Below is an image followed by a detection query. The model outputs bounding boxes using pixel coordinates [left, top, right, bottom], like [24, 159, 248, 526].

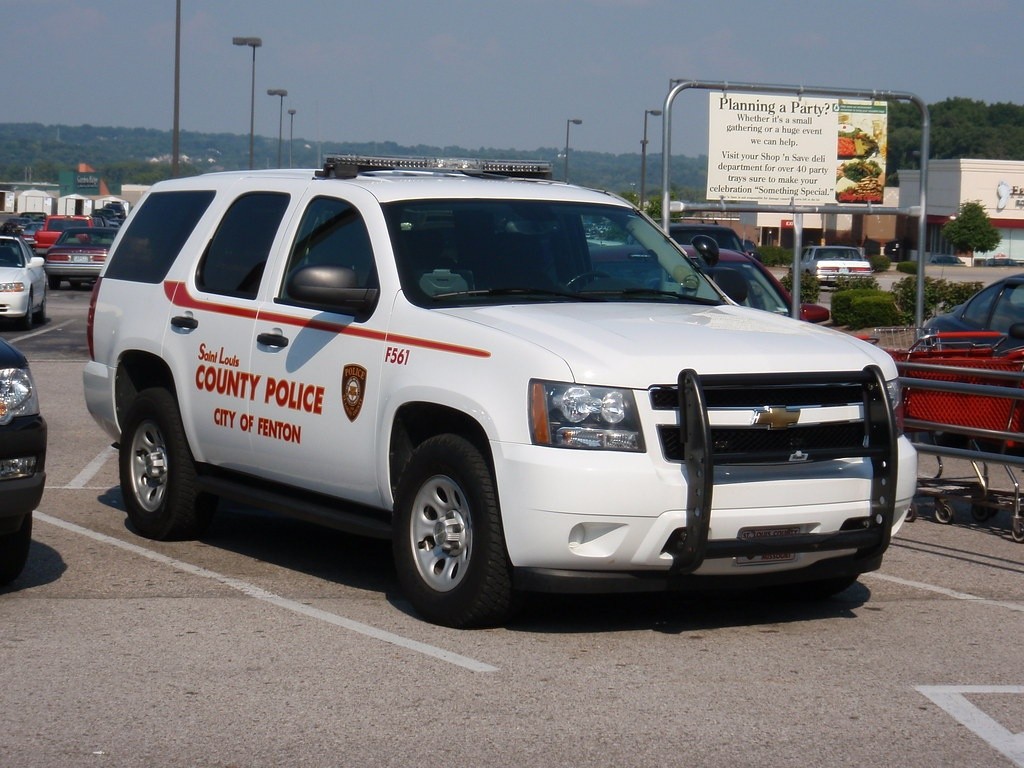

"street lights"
[564, 119, 582, 182]
[288, 109, 297, 169]
[640, 109, 662, 211]
[233, 37, 262, 170]
[267, 89, 288, 169]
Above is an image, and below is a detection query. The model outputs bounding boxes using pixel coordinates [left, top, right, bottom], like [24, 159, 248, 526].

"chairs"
[495, 234, 577, 293]
[67, 238, 81, 244]
[0, 247, 16, 261]
[366, 230, 476, 295]
[101, 239, 113, 244]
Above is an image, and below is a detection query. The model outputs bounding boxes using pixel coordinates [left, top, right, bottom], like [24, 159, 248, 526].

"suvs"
[83, 162, 917, 629]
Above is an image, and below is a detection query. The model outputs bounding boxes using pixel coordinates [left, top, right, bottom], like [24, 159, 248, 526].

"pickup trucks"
[32, 215, 93, 254]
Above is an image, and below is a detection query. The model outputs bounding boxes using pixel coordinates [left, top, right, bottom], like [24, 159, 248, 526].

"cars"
[0, 337, 48, 584]
[924, 273, 1024, 357]
[89, 203, 127, 228]
[929, 255, 967, 267]
[984, 259, 1017, 267]
[789, 246, 873, 288]
[624, 224, 755, 257]
[44, 227, 119, 290]
[0, 236, 49, 330]
[588, 244, 830, 323]
[0, 212, 48, 244]
